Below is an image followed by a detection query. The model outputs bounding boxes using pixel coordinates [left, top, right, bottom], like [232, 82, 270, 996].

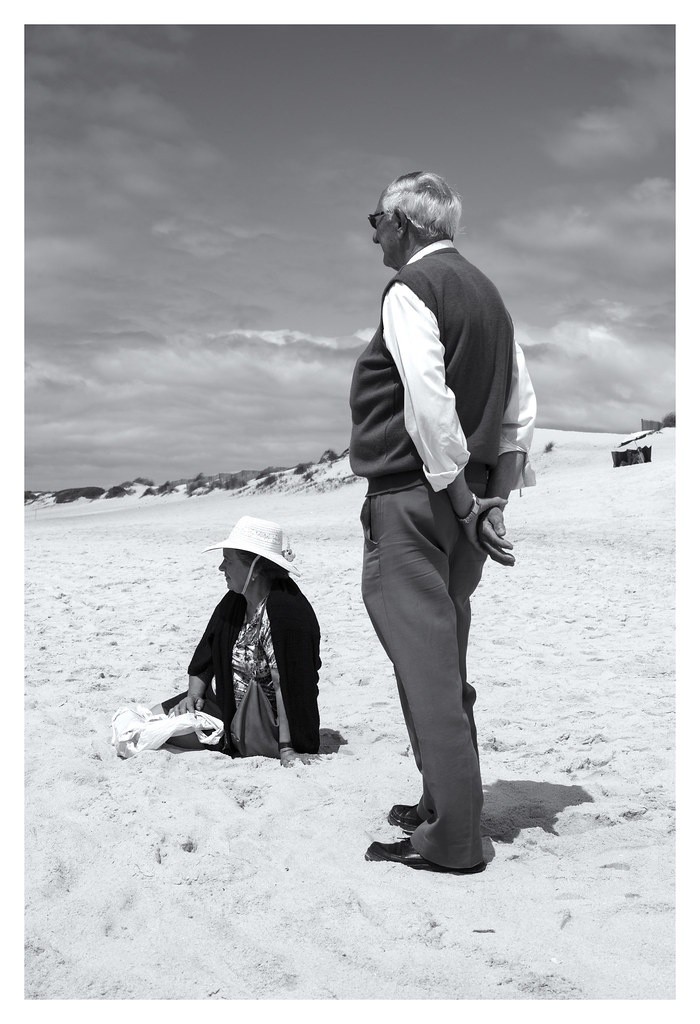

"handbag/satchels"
[231, 679, 281, 758]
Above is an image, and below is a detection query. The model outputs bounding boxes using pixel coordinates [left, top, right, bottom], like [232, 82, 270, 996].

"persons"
[151, 515, 322, 769]
[348, 168, 537, 875]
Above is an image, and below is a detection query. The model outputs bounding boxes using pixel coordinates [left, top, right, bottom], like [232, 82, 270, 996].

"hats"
[201, 514, 302, 577]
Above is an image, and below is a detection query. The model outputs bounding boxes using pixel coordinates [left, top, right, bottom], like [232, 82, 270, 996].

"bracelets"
[278, 742, 294, 753]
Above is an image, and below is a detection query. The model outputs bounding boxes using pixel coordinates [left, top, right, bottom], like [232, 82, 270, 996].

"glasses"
[366, 211, 384, 229]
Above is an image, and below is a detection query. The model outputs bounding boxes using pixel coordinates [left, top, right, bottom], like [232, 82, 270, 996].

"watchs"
[458, 490, 481, 527]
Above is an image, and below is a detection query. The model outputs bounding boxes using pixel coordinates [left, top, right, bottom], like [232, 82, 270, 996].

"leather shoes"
[388, 804, 424, 832]
[366, 836, 486, 875]
